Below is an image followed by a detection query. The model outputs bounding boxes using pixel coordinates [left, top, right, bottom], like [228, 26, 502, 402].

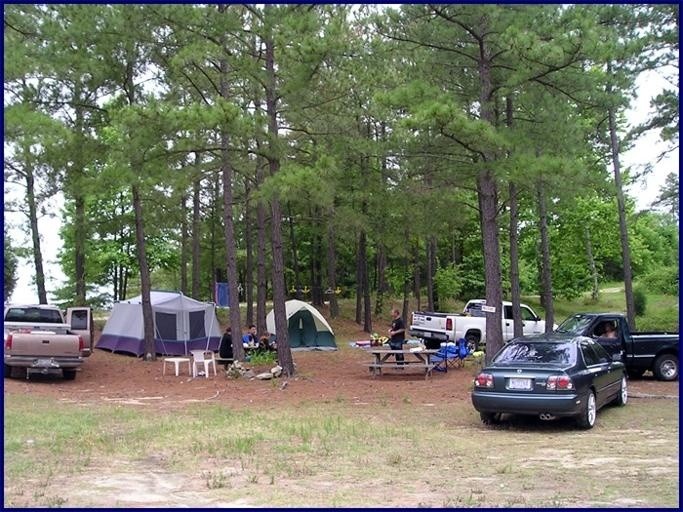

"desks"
[366, 349, 440, 377]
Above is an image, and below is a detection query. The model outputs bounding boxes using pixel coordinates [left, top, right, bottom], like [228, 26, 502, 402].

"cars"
[471, 332, 630, 429]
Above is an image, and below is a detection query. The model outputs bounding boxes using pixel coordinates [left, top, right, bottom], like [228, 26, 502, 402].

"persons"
[592, 322, 618, 338]
[242, 325, 278, 353]
[219, 327, 234, 371]
[388, 309, 406, 370]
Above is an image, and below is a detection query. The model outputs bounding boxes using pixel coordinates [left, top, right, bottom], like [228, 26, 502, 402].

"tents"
[94, 290, 223, 358]
[266, 299, 338, 352]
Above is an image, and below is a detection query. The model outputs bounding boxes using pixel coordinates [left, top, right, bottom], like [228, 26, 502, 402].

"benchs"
[214, 357, 233, 370]
[362, 360, 436, 381]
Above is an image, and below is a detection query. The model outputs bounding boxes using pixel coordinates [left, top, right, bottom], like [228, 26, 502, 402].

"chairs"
[188, 349, 217, 379]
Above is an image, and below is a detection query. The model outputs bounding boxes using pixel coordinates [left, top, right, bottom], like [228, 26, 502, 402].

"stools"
[162, 357, 190, 376]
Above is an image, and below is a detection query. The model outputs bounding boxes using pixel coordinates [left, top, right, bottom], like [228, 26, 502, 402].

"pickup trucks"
[408, 297, 560, 354]
[543, 311, 680, 382]
[3, 303, 94, 382]
